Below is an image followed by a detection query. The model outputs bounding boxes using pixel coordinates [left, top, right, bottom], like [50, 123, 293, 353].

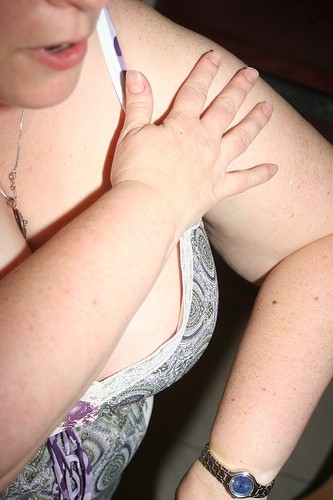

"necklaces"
[0, 109, 27, 241]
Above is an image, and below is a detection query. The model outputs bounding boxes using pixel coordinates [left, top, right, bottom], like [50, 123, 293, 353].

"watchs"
[197, 443, 276, 500]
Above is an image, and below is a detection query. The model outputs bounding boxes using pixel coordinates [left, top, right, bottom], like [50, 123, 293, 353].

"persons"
[0, 1, 332, 500]
